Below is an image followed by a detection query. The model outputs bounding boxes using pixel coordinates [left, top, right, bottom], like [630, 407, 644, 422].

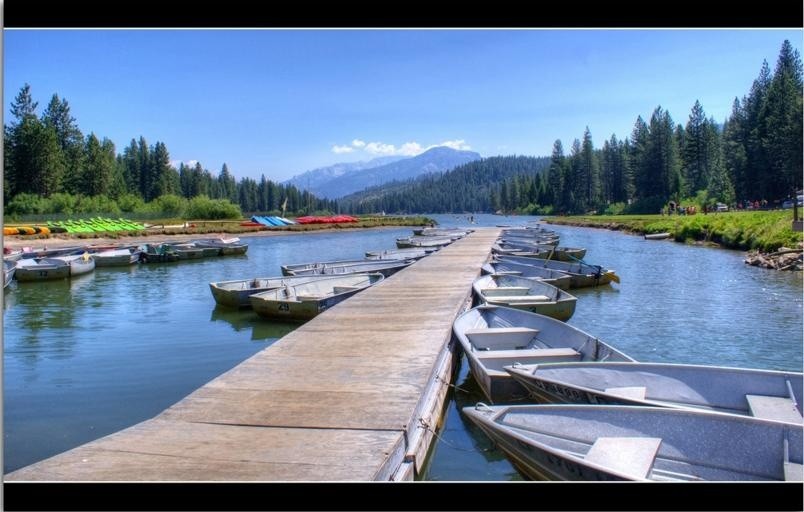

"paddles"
[567, 253, 620, 284]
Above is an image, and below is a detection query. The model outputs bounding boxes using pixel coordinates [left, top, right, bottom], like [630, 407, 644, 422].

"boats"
[1, 236, 249, 290]
[4, 216, 146, 238]
[249, 214, 358, 228]
[209, 226, 475, 320]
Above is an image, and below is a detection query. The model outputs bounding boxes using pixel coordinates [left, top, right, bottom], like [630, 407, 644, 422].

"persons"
[660, 198, 770, 217]
